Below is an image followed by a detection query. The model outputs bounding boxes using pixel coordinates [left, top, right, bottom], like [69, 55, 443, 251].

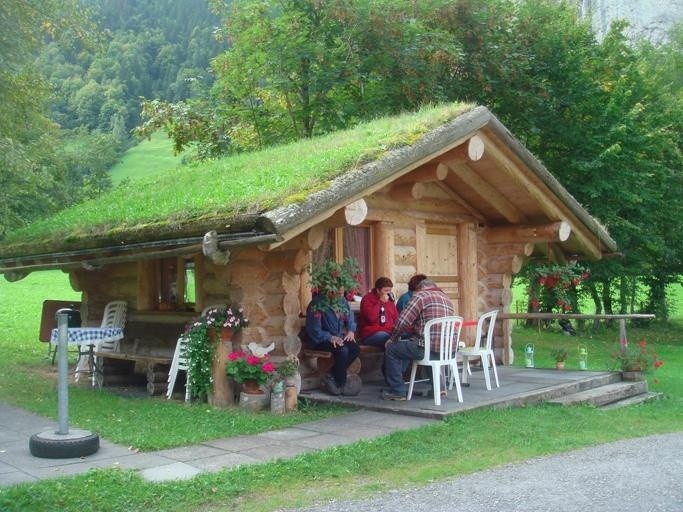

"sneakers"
[382, 390, 407, 401]
[427, 390, 447, 399]
[320, 373, 344, 394]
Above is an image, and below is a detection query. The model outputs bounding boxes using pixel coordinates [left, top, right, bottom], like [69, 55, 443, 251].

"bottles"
[378, 303, 385, 326]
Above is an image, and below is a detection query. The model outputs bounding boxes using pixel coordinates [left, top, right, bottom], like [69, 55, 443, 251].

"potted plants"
[549, 347, 569, 370]
[276, 356, 304, 387]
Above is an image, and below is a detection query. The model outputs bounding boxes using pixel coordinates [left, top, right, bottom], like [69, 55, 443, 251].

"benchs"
[302, 344, 384, 360]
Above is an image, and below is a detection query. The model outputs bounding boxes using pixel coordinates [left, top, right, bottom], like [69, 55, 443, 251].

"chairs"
[406, 315, 465, 407]
[164, 302, 228, 403]
[447, 309, 501, 392]
[73, 299, 129, 387]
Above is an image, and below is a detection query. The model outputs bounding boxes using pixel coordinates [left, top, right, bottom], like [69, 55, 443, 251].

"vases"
[207, 327, 235, 341]
[620, 370, 645, 382]
[241, 379, 260, 394]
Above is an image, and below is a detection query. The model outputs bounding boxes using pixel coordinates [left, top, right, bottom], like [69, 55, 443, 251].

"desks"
[49, 327, 126, 374]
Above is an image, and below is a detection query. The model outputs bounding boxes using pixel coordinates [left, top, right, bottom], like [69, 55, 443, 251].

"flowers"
[608, 338, 663, 386]
[301, 254, 365, 321]
[224, 351, 275, 386]
[177, 302, 251, 406]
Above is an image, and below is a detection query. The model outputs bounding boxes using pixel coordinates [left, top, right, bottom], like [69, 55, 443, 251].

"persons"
[359, 277, 415, 386]
[305, 284, 360, 395]
[396, 275, 427, 341]
[382, 279, 457, 401]
